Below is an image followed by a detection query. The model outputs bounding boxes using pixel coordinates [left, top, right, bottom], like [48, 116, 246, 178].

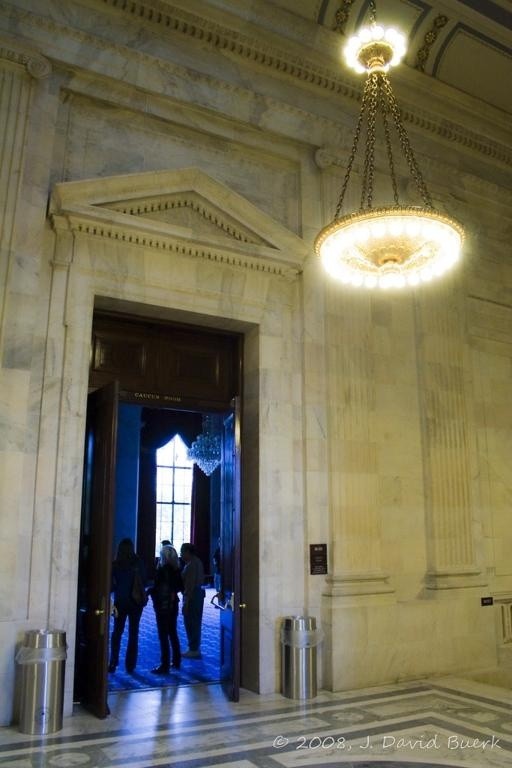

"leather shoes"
[152, 666, 169, 673]
[110, 663, 115, 671]
[125, 662, 135, 671]
[170, 662, 180, 669]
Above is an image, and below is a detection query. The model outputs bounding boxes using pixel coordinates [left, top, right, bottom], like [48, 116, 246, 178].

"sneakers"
[182, 650, 201, 657]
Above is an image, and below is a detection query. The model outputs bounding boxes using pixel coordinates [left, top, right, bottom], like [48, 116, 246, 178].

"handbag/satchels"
[132, 582, 143, 600]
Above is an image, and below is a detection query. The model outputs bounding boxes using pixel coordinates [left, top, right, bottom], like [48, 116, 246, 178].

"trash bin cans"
[16, 629, 66, 735]
[282, 616, 318, 700]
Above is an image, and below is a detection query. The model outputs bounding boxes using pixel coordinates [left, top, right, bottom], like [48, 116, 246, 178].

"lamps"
[187, 413, 220, 476]
[308, 0, 470, 294]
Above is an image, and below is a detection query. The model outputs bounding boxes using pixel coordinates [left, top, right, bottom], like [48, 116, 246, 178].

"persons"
[180, 543, 204, 659]
[156, 540, 173, 570]
[110, 538, 149, 673]
[147, 545, 184, 673]
